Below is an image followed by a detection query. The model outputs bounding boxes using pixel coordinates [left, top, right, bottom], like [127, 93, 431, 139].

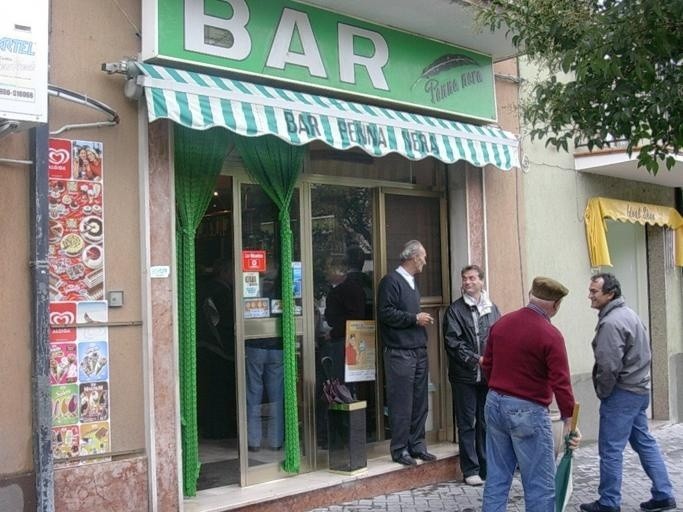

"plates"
[47, 178, 103, 301]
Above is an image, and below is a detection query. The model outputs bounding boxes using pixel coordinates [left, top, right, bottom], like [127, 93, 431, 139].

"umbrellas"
[552, 400, 580, 512]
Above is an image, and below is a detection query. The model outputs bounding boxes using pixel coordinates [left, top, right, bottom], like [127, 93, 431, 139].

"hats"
[532, 277, 568, 300]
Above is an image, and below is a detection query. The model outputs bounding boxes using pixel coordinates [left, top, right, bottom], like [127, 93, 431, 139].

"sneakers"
[580, 501, 619, 512]
[640, 499, 676, 511]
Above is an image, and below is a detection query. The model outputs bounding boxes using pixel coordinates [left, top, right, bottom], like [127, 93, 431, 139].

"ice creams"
[79, 344, 107, 377]
[48, 347, 74, 384]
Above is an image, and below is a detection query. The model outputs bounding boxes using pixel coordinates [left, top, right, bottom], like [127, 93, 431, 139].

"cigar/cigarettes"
[430, 320, 433, 325]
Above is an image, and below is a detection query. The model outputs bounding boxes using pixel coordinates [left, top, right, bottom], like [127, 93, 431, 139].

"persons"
[482, 276, 582, 512]
[207, 246, 374, 452]
[441, 264, 503, 486]
[376, 239, 437, 467]
[579, 272, 677, 512]
[73, 141, 101, 181]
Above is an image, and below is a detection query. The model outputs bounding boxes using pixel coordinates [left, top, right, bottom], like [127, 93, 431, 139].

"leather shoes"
[399, 456, 416, 465]
[464, 475, 482, 485]
[419, 453, 435, 461]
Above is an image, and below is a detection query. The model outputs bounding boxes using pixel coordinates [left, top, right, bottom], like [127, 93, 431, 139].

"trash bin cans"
[327, 399, 368, 476]
